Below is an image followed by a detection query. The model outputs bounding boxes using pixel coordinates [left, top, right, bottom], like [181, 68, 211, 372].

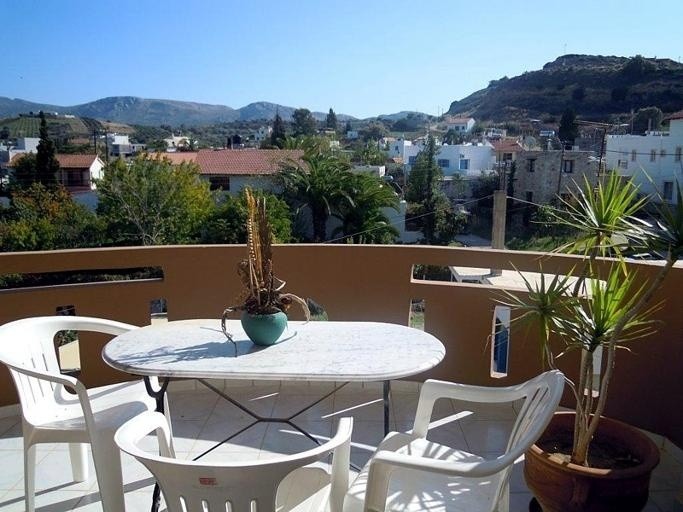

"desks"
[102, 318, 446, 512]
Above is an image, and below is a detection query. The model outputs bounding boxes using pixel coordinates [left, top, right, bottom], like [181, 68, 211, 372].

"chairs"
[0, 315, 176, 512]
[343, 370, 564, 512]
[113, 410, 353, 512]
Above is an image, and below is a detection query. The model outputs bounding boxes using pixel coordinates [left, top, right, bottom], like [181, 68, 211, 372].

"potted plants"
[222, 188, 310, 357]
[488, 161, 683, 512]
